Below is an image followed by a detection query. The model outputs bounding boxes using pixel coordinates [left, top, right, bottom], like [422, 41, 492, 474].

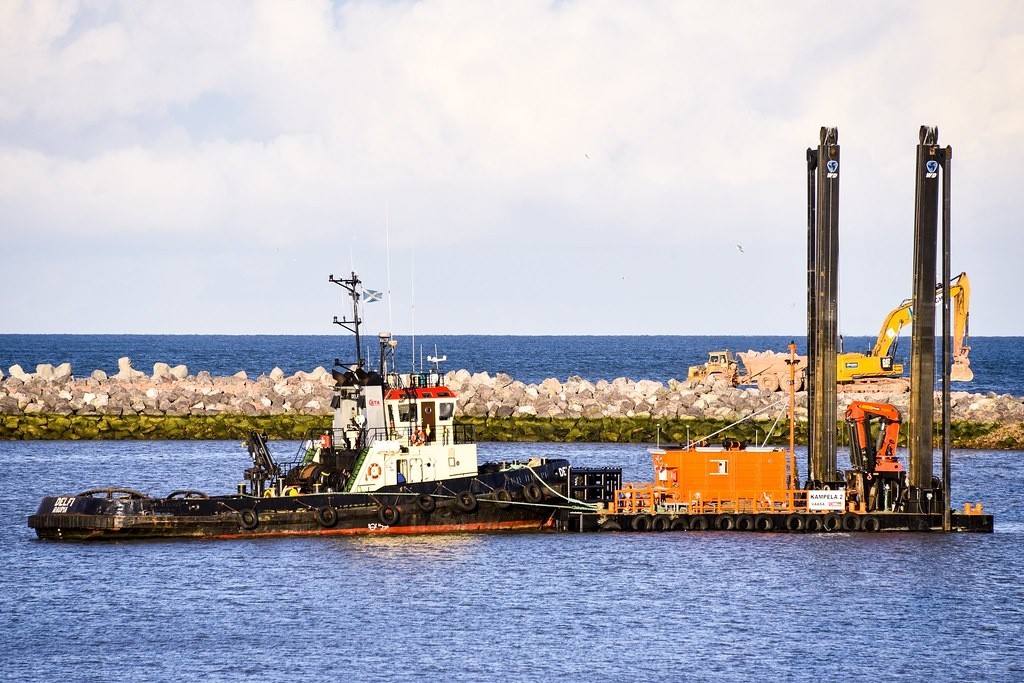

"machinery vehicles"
[836, 271, 974, 393]
[844, 400, 905, 505]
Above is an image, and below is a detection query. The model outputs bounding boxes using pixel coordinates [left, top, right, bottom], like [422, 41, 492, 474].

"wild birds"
[736, 243, 744, 254]
[585, 154, 589, 158]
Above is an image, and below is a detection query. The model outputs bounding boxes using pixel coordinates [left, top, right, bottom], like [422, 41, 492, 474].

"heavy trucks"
[687, 348, 807, 392]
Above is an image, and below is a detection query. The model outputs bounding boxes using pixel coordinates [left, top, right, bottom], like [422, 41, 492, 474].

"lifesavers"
[367, 463, 382, 479]
[410, 429, 426, 446]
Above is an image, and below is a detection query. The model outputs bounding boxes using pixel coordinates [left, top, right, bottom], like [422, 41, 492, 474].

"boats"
[24, 212, 574, 542]
